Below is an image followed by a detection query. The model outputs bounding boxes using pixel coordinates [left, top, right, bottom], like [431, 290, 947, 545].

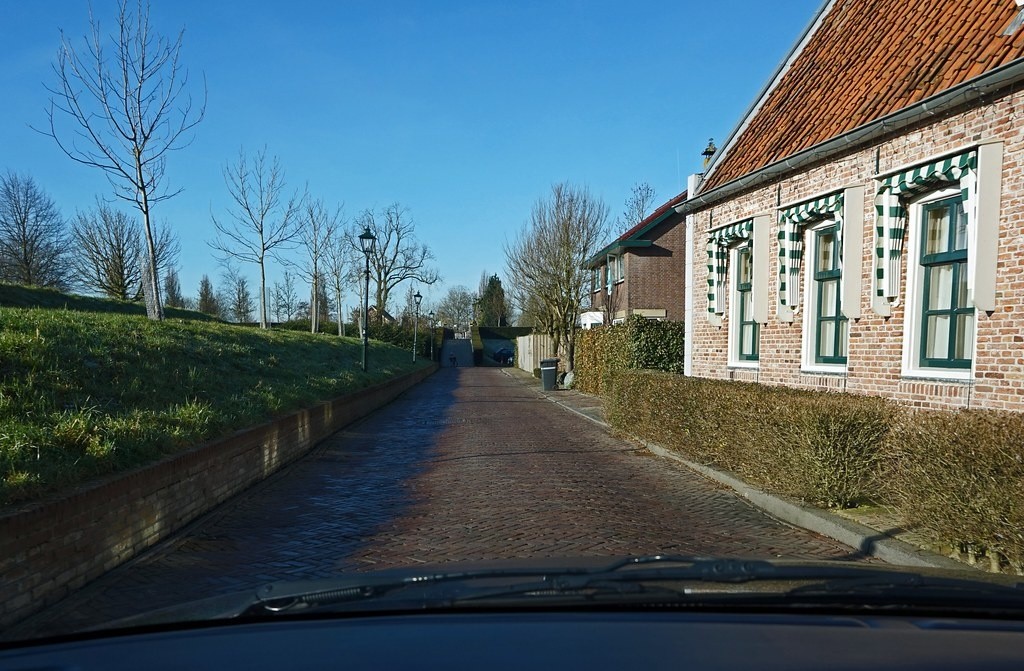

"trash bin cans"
[540, 358, 561, 391]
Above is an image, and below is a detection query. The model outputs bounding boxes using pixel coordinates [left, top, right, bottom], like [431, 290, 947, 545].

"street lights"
[412, 290, 422, 362]
[429, 310, 435, 361]
[358, 225, 376, 372]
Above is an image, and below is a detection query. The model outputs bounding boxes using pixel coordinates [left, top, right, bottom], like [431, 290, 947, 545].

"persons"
[448, 328, 454, 339]
[449, 353, 458, 368]
